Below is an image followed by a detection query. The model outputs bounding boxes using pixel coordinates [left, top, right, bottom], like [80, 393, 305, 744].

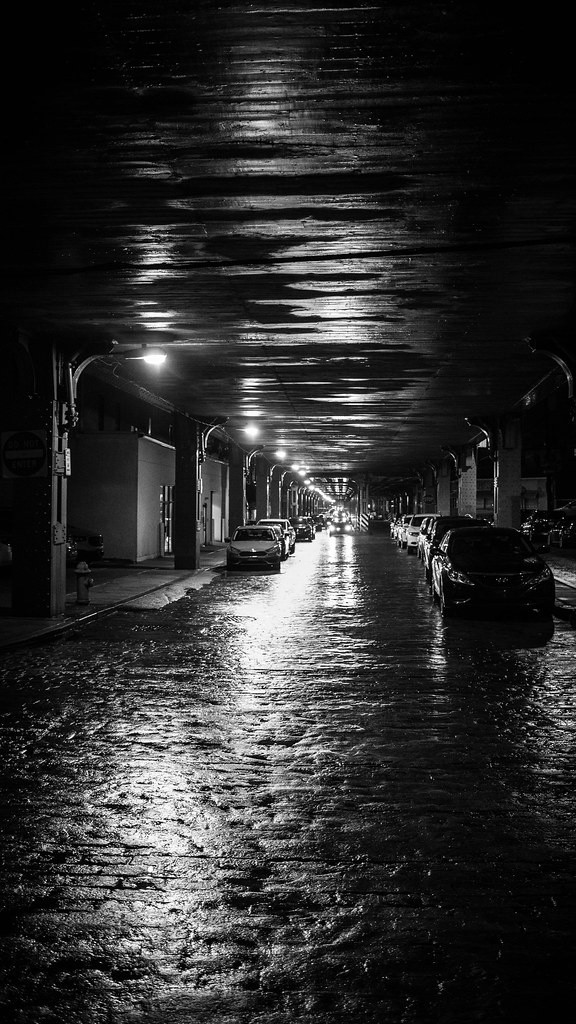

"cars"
[224, 525, 284, 571]
[289, 514, 328, 542]
[66, 524, 105, 567]
[256, 518, 298, 555]
[273, 524, 290, 561]
[430, 525, 556, 620]
[520, 500, 576, 547]
[388, 513, 493, 586]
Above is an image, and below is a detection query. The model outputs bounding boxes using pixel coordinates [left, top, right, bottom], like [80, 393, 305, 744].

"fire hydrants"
[74, 561, 94, 604]
